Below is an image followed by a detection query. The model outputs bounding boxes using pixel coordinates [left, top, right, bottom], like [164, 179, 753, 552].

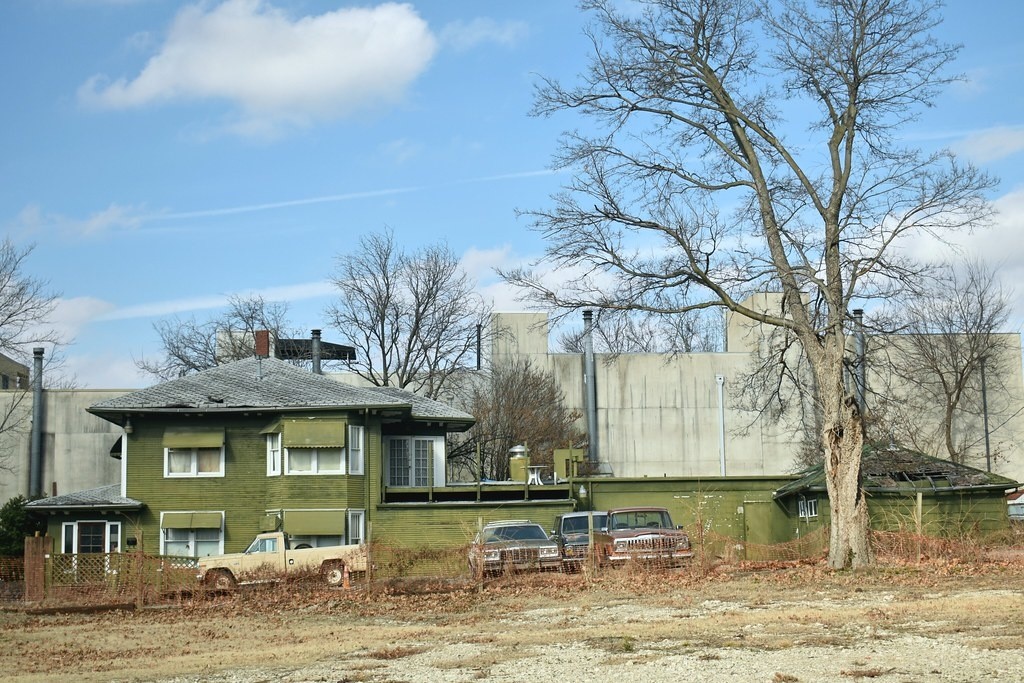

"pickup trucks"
[198, 531, 370, 594]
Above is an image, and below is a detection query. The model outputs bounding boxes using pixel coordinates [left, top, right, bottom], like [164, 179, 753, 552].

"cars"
[601, 507, 693, 566]
[550, 511, 607, 573]
[468, 518, 563, 578]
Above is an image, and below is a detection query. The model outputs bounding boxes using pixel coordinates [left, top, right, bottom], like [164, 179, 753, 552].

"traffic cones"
[341, 565, 352, 594]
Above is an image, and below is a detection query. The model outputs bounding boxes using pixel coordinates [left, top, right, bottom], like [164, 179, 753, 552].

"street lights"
[979, 353, 992, 473]
